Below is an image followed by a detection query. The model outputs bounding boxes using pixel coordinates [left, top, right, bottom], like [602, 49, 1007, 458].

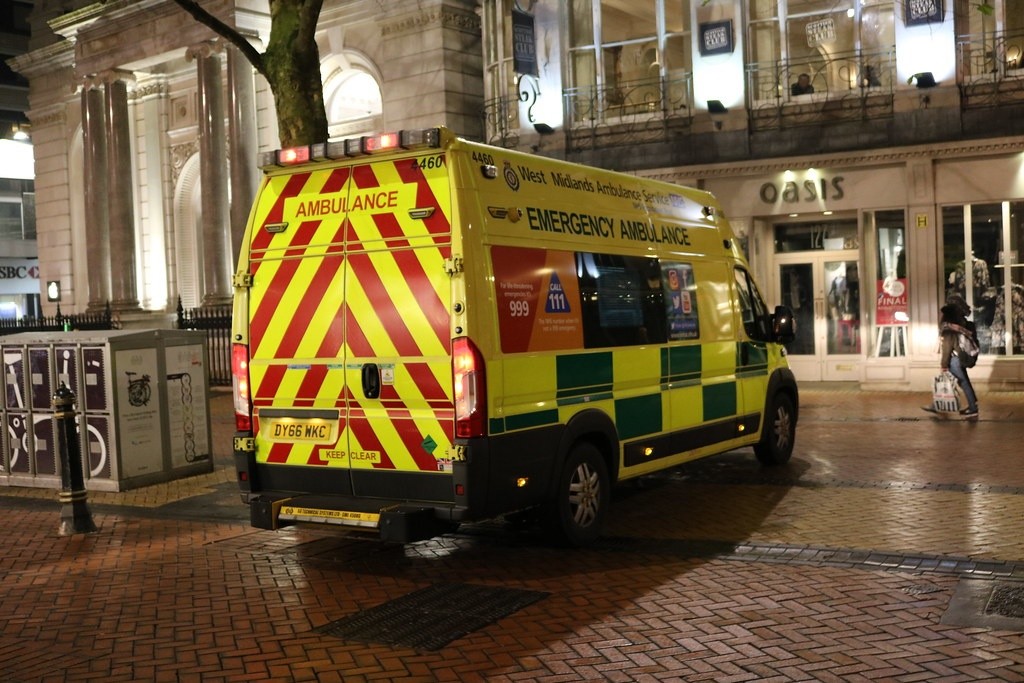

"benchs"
[989, 360, 1024, 390]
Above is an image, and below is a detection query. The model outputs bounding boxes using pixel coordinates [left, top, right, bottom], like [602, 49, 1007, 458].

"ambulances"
[233, 125, 800, 544]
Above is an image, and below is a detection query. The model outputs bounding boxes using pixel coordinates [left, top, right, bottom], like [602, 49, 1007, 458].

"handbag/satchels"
[932, 372, 960, 412]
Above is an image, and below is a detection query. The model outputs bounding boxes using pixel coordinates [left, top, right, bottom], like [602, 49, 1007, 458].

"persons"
[862, 65, 879, 89]
[828, 274, 846, 338]
[790, 73, 814, 95]
[922, 294, 981, 419]
[948, 257, 989, 307]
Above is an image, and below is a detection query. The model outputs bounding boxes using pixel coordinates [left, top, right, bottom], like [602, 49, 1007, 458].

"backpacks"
[950, 321, 980, 368]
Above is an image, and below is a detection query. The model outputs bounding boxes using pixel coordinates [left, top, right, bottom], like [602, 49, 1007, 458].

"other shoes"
[959, 405, 979, 417]
[920, 404, 939, 413]
